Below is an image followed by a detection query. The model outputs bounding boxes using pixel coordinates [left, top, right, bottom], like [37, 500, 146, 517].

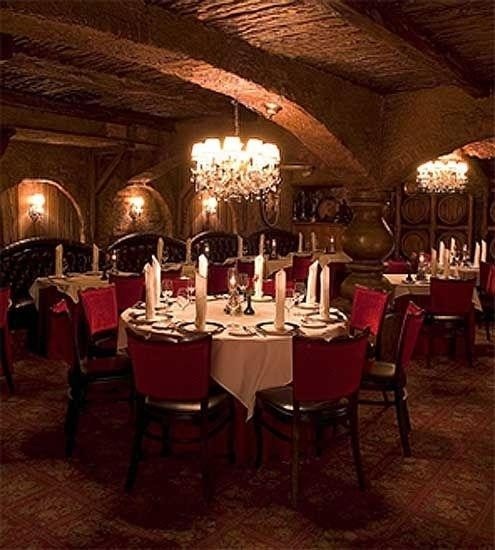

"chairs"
[2, 246, 489, 396]
[114, 326, 241, 497]
[343, 300, 428, 461]
[251, 325, 374, 509]
[59, 280, 139, 452]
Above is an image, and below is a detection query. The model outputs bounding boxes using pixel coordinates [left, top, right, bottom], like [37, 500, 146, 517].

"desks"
[115, 291, 355, 474]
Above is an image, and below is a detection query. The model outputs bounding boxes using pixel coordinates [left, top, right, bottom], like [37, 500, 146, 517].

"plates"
[128, 293, 343, 335]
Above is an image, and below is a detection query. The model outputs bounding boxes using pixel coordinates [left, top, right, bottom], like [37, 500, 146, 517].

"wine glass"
[160, 268, 307, 325]
[416, 243, 471, 279]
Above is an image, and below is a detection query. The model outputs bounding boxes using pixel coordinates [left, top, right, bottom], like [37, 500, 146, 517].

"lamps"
[415, 151, 468, 196]
[190, 98, 280, 204]
